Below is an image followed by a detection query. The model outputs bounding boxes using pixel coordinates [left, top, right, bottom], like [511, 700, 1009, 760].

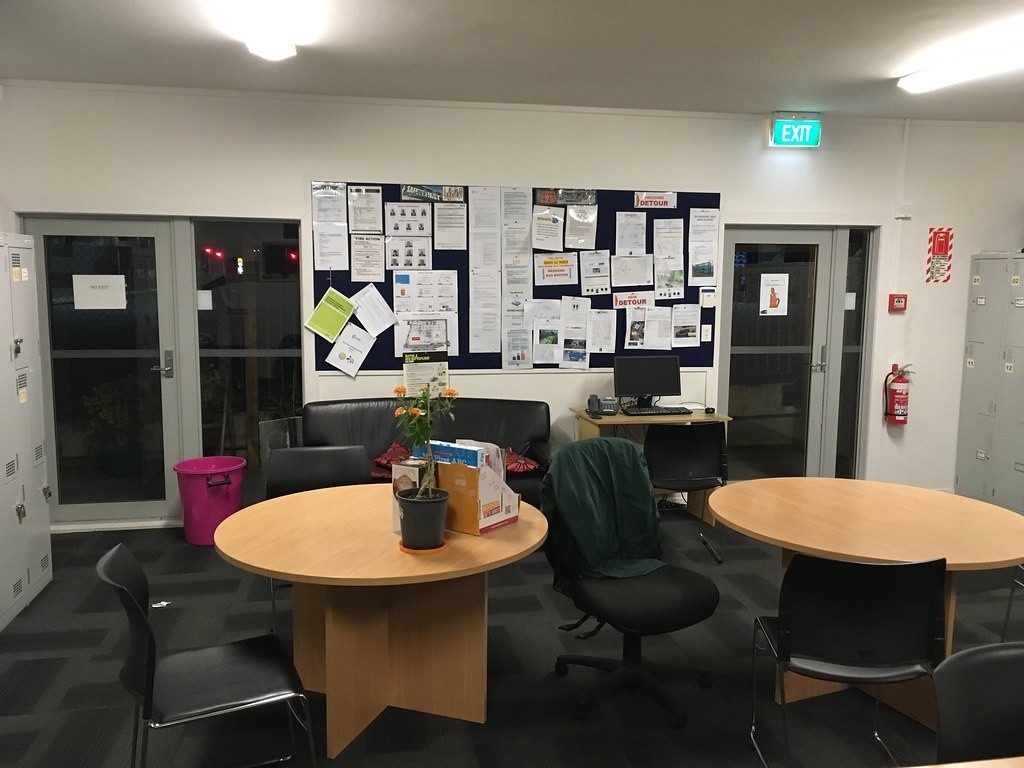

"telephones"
[588, 394, 619, 416]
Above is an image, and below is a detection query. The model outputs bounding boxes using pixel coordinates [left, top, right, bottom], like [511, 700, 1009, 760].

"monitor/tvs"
[614, 356, 681, 406]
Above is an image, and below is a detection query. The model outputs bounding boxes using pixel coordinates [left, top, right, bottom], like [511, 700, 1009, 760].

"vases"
[395, 487, 451, 554]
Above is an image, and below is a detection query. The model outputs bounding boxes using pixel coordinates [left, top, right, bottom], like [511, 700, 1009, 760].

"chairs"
[934, 641, 1024, 763]
[640, 420, 725, 565]
[95, 542, 318, 768]
[263, 445, 372, 499]
[544, 438, 719, 729]
[749, 554, 947, 768]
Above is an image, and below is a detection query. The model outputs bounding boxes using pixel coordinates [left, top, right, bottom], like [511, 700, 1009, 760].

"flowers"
[394, 382, 458, 500]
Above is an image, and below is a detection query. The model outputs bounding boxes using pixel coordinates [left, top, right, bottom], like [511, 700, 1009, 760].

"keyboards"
[621, 407, 693, 416]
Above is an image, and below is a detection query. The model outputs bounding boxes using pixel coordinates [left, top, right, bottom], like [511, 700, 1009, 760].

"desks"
[569, 407, 733, 526]
[213, 483, 551, 759]
[710, 476, 1024, 737]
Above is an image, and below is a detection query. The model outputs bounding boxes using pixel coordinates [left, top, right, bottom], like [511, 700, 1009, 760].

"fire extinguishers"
[884, 364, 915, 425]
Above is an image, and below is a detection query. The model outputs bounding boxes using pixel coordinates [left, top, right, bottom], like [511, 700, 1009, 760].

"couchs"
[303, 397, 550, 511]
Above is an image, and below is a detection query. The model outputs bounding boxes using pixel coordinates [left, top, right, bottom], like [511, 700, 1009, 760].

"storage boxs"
[433, 459, 521, 535]
[392, 458, 431, 532]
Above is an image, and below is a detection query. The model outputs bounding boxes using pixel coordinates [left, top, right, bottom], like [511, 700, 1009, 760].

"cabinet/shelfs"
[0, 232, 53, 635]
[955, 254, 1024, 517]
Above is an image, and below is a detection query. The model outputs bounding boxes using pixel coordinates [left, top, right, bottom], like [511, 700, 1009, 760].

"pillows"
[375, 441, 411, 467]
[503, 449, 546, 472]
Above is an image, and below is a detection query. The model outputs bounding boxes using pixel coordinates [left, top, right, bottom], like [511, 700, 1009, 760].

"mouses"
[705, 408, 715, 414]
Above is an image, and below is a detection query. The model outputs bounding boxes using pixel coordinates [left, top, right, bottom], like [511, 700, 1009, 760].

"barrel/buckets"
[172, 457, 247, 545]
